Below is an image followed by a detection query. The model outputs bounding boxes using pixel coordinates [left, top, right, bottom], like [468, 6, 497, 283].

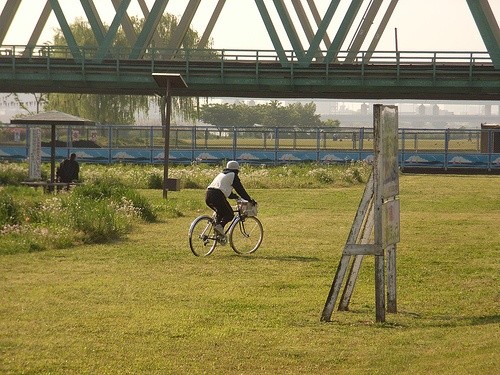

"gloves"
[234, 196, 239, 200]
[249, 199, 257, 207]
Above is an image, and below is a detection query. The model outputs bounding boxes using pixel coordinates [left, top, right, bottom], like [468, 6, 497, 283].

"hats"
[226, 161, 239, 169]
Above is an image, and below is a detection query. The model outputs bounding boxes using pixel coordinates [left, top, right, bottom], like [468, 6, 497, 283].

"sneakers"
[214, 224, 225, 235]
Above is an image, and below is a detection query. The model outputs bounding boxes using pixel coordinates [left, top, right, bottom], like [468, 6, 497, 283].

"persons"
[205, 161, 257, 237]
[57, 154, 79, 183]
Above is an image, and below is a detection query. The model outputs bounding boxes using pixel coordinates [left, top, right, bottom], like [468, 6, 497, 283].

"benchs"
[21, 181, 85, 196]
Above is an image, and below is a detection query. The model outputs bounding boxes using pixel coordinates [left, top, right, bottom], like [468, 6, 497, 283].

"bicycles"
[188, 197, 264, 257]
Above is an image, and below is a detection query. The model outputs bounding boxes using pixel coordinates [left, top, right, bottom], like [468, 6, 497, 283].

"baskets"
[237, 198, 257, 217]
